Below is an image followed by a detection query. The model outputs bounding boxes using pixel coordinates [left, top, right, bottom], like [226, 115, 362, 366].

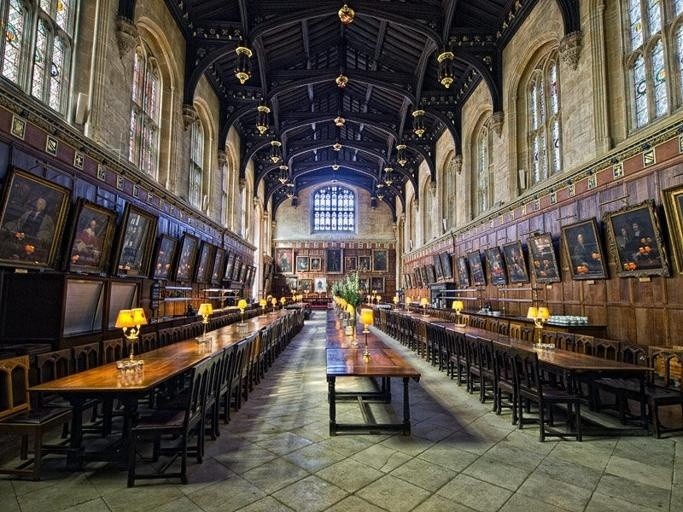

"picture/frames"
[1, 163, 683, 294]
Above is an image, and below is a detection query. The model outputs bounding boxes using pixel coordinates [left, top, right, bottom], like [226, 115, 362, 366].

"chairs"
[0, 291, 683, 489]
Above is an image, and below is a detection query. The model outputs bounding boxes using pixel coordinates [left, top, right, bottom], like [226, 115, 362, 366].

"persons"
[630, 220, 657, 250]
[3, 198, 56, 260]
[72, 218, 103, 267]
[571, 233, 600, 267]
[276, 249, 388, 296]
[618, 225, 635, 259]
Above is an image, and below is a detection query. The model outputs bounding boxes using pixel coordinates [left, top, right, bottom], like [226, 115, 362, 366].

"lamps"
[233, 3, 455, 202]
